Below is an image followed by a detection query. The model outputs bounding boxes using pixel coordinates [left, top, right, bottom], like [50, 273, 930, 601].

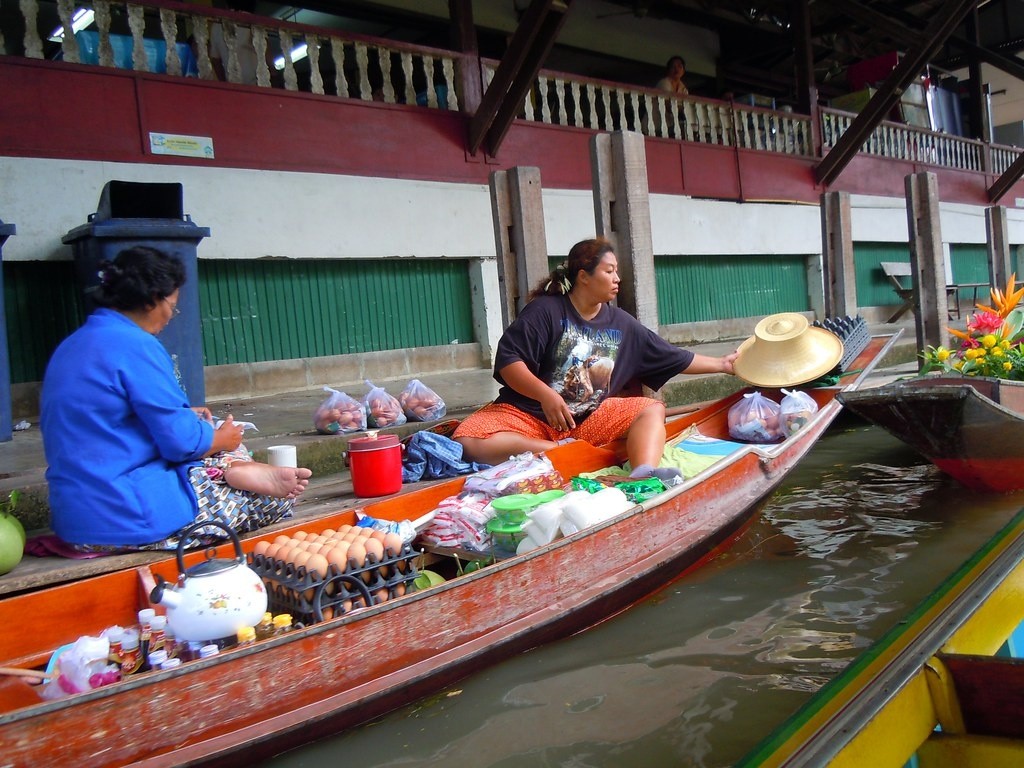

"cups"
[267, 445, 297, 504]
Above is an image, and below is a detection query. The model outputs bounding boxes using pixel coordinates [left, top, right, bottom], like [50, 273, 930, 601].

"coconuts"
[0, 510, 27, 575]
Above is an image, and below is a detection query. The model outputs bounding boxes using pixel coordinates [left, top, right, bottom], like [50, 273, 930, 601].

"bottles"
[105, 605, 294, 672]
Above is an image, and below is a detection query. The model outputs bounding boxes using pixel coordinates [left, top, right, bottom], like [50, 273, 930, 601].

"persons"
[39, 243, 311, 552]
[451, 238, 739, 480]
[642, 55, 690, 134]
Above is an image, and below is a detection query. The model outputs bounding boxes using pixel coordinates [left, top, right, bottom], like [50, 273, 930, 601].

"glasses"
[162, 296, 181, 321]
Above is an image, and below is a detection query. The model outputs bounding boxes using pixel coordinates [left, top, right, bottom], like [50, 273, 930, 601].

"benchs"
[879, 260, 960, 326]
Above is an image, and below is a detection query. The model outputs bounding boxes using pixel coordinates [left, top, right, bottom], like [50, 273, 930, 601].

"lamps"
[270, 13, 324, 71]
[43, 6, 96, 44]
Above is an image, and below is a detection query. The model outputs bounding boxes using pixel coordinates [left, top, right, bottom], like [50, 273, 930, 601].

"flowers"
[906, 269, 1024, 381]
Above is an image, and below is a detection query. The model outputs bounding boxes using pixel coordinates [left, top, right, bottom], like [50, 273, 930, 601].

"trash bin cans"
[0, 220, 17, 444]
[61, 180, 211, 412]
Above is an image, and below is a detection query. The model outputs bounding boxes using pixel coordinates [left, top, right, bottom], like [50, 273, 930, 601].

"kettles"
[150, 520, 268, 642]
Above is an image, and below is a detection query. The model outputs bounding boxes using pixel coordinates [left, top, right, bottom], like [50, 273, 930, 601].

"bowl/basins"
[486, 490, 567, 553]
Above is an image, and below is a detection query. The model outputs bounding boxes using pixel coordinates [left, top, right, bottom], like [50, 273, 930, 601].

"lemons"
[939, 335, 1012, 371]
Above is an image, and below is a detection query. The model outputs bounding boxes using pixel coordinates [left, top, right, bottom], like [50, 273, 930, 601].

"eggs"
[315, 392, 439, 433]
[728, 406, 783, 438]
[254, 524, 406, 623]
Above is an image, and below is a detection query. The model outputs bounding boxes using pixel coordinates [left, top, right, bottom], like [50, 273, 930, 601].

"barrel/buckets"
[342, 430, 405, 499]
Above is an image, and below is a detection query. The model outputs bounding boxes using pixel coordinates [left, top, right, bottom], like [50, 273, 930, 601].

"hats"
[730, 312, 845, 388]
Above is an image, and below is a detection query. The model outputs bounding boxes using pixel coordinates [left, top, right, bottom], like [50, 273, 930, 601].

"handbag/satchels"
[780, 389, 819, 439]
[362, 380, 406, 428]
[311, 386, 368, 435]
[397, 379, 447, 422]
[726, 391, 783, 442]
